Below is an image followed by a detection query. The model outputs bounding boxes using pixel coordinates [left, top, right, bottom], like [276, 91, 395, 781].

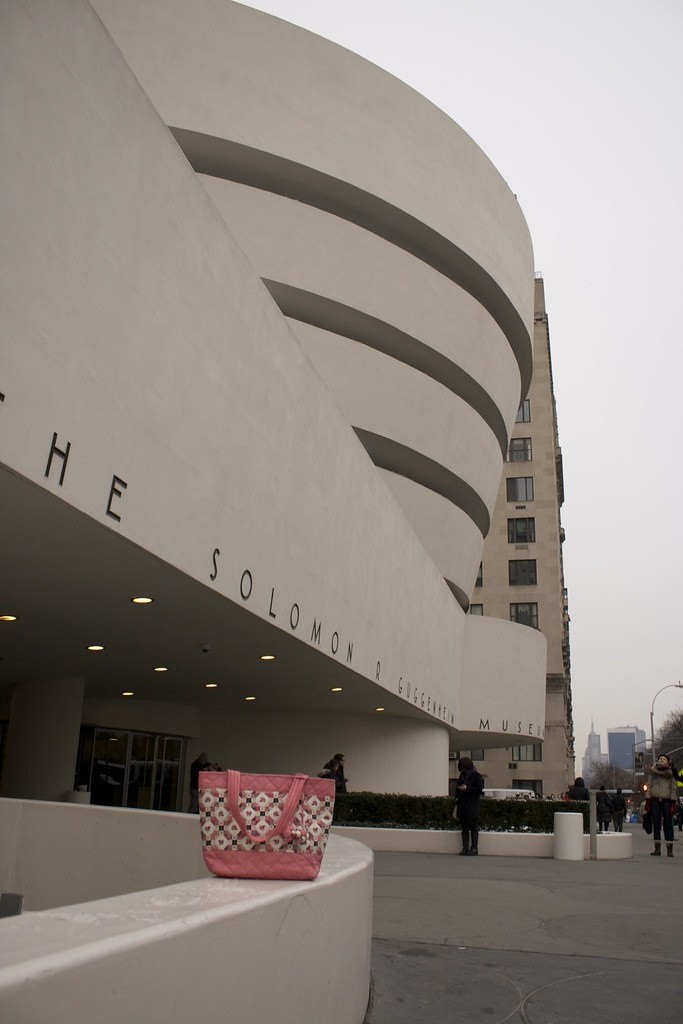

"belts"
[650, 796, 670, 802]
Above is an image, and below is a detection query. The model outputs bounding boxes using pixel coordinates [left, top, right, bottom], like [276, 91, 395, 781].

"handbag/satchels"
[642, 812, 652, 834]
[197, 768, 336, 882]
[452, 803, 460, 821]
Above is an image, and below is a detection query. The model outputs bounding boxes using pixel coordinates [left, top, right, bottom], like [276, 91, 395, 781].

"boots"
[650, 840, 661, 856]
[666, 841, 674, 857]
[459, 847, 478, 856]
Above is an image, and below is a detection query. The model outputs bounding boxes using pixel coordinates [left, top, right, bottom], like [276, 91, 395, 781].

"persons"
[189, 753, 211, 814]
[569, 777, 589, 800]
[612, 788, 627, 832]
[455, 757, 483, 856]
[623, 800, 647, 830]
[596, 786, 613, 830]
[515, 791, 570, 801]
[317, 754, 349, 793]
[645, 755, 678, 857]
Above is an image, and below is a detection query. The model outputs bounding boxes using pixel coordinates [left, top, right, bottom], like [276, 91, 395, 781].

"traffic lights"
[643, 784, 648, 791]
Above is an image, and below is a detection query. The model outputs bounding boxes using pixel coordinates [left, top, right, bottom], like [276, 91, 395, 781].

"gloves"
[645, 799, 650, 811]
[671, 801, 676, 816]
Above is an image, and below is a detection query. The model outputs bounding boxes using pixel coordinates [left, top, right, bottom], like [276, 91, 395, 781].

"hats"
[658, 755, 670, 761]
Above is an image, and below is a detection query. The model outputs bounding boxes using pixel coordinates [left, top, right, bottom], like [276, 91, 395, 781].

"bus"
[481, 788, 536, 801]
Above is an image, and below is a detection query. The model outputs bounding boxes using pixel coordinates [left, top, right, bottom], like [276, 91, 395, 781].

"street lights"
[630, 738, 660, 773]
[648, 681, 683, 762]
